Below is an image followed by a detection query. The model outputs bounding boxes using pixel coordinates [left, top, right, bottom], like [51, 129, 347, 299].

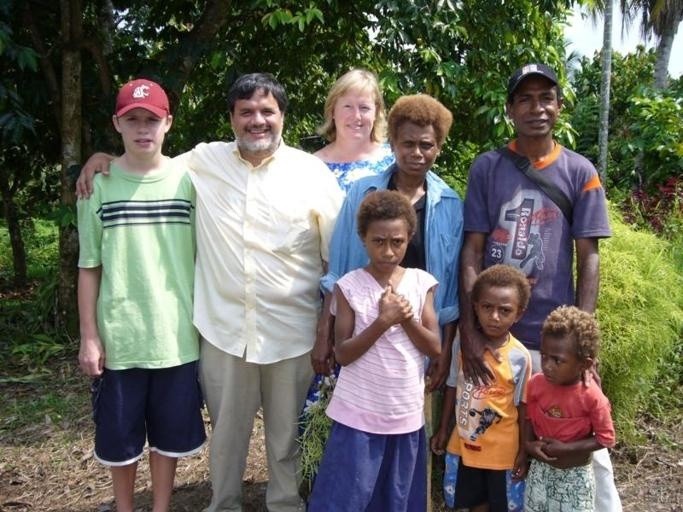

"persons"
[313, 91, 465, 510]
[76, 63, 345, 511]
[456, 63, 622, 512]
[305, 189, 441, 511]
[431, 263, 617, 511]
[301, 71, 392, 441]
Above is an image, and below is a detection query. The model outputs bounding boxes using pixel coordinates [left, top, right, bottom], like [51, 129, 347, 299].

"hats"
[114, 78, 170, 119]
[508, 63, 558, 96]
